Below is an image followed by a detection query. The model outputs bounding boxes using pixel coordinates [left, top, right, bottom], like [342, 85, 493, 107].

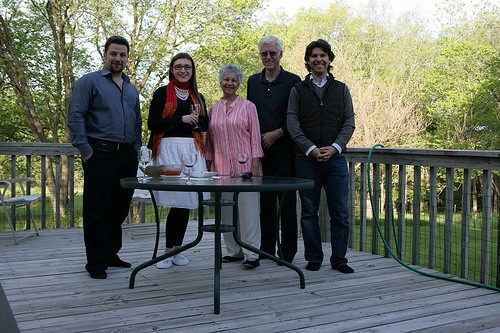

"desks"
[121, 175, 315, 315]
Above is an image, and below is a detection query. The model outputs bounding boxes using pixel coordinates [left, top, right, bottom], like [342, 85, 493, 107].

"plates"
[161, 175, 182, 178]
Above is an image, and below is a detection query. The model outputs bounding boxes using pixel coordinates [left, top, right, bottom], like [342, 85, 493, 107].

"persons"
[286, 38, 356, 274]
[246, 35, 302, 264]
[68, 35, 143, 280]
[204, 63, 264, 268]
[147, 53, 210, 269]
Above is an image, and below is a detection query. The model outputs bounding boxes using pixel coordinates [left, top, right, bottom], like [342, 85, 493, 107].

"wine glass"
[182, 152, 197, 184]
[190, 104, 201, 129]
[236, 152, 249, 176]
[137, 149, 152, 182]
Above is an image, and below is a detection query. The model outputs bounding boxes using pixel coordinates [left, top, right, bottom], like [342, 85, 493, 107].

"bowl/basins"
[162, 168, 181, 175]
[140, 166, 173, 176]
[184, 171, 218, 177]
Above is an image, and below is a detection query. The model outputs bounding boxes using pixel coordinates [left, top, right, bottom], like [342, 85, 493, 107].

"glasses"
[171, 65, 193, 71]
[260, 51, 279, 56]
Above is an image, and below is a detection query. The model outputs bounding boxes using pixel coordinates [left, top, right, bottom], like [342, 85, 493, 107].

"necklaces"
[174, 85, 190, 101]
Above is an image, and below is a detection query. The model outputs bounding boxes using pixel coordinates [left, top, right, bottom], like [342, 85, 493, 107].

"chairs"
[0, 177, 43, 245]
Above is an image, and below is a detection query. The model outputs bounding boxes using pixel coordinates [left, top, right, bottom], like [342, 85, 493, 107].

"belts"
[89, 138, 126, 151]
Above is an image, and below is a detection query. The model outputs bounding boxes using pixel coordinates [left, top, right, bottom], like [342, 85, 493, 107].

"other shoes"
[332, 264, 354, 273]
[222, 255, 244, 261]
[306, 261, 321, 271]
[278, 255, 293, 266]
[171, 254, 189, 266]
[156, 258, 172, 268]
[243, 260, 260, 268]
[107, 259, 131, 268]
[85, 265, 107, 280]
